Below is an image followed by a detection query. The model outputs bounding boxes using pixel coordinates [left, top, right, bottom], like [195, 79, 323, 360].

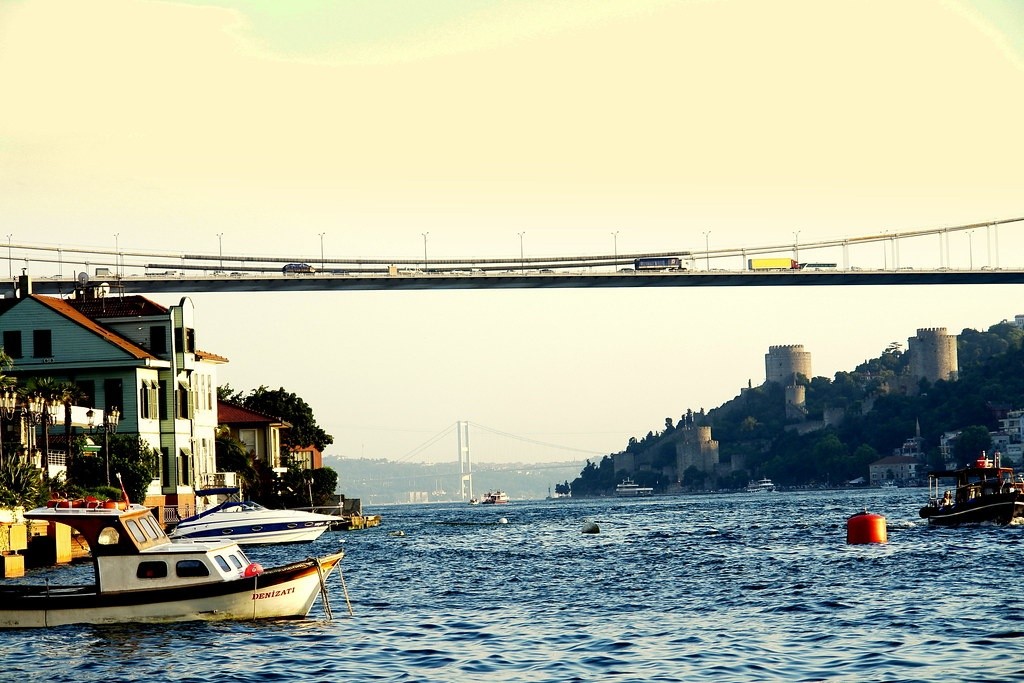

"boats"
[468, 489, 509, 505]
[745, 476, 776, 495]
[0, 464, 346, 629]
[164, 484, 345, 547]
[614, 476, 654, 498]
[918, 450, 1024, 528]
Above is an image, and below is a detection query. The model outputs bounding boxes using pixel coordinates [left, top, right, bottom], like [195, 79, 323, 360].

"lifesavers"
[46, 499, 83, 508]
[85, 499, 127, 510]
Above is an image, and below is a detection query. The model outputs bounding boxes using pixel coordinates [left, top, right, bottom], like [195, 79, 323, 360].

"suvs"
[282, 263, 315, 276]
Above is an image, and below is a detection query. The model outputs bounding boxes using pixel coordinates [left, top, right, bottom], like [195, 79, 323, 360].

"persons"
[941, 491, 952, 507]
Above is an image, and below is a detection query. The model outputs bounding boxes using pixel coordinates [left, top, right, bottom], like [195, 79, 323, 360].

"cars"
[399, 268, 558, 278]
[52, 269, 241, 280]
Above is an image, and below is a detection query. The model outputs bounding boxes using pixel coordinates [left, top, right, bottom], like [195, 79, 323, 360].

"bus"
[801, 263, 838, 272]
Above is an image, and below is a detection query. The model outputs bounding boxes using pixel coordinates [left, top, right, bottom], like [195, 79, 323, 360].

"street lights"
[702, 231, 711, 272]
[114, 233, 119, 275]
[421, 232, 429, 271]
[518, 232, 526, 273]
[793, 230, 802, 263]
[28, 391, 63, 492]
[6, 234, 14, 278]
[0, 384, 19, 492]
[318, 232, 326, 274]
[880, 230, 890, 269]
[611, 230, 620, 274]
[86, 405, 121, 486]
[217, 233, 224, 266]
[966, 230, 975, 270]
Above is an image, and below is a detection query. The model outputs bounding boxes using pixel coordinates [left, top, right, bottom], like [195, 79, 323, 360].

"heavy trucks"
[618, 256, 692, 274]
[748, 257, 798, 272]
